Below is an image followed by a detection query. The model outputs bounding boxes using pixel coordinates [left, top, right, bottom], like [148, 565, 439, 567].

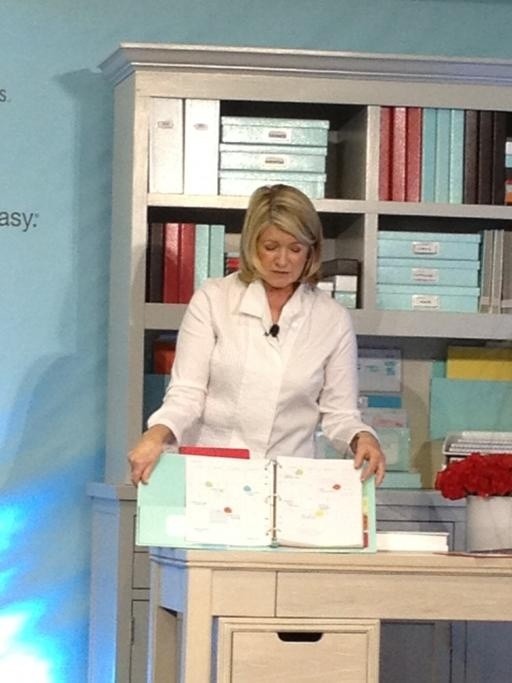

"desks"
[146, 546, 512, 682]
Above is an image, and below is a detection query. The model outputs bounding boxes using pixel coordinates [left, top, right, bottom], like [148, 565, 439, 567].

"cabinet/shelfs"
[86, 41, 512, 683]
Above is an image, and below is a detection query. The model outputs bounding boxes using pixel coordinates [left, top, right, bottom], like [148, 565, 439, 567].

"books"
[373, 527, 450, 554]
[145, 219, 365, 313]
[376, 104, 511, 316]
[448, 447, 512, 453]
[451, 437, 512, 443]
[448, 443, 512, 450]
[446, 429, 512, 435]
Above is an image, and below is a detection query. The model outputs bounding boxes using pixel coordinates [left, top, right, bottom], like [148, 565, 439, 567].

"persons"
[126, 180, 388, 491]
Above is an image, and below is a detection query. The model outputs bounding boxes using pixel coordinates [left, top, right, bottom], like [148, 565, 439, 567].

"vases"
[464, 494, 512, 552]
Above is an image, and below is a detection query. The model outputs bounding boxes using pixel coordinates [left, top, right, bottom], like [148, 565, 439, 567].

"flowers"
[434, 453, 512, 502]
